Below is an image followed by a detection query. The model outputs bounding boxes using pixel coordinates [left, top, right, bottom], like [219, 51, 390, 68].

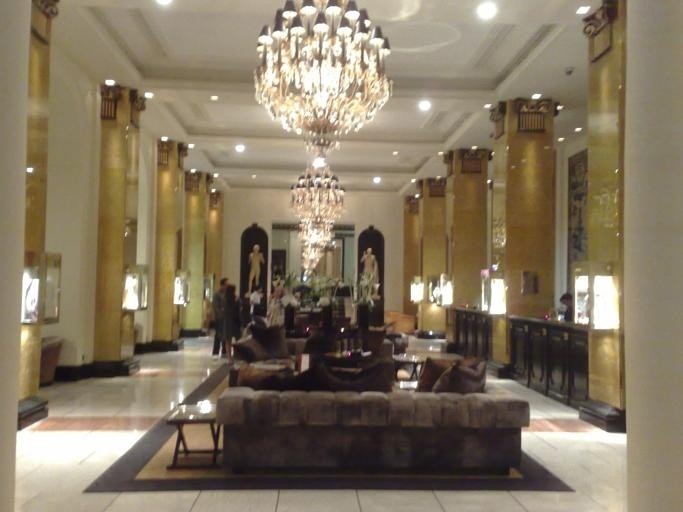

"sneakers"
[213, 353, 227, 360]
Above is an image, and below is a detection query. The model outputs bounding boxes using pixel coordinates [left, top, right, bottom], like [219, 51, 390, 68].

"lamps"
[252, 0, 394, 275]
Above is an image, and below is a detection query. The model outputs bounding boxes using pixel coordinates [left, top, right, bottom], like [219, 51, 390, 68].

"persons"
[245, 244, 265, 298]
[560, 294, 571, 322]
[250, 286, 265, 314]
[223, 285, 241, 364]
[360, 248, 381, 300]
[211, 278, 228, 360]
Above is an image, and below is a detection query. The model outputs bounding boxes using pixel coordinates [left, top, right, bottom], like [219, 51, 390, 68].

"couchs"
[218, 318, 530, 477]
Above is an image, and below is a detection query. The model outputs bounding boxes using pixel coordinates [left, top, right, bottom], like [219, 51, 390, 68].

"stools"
[166, 402, 221, 472]
